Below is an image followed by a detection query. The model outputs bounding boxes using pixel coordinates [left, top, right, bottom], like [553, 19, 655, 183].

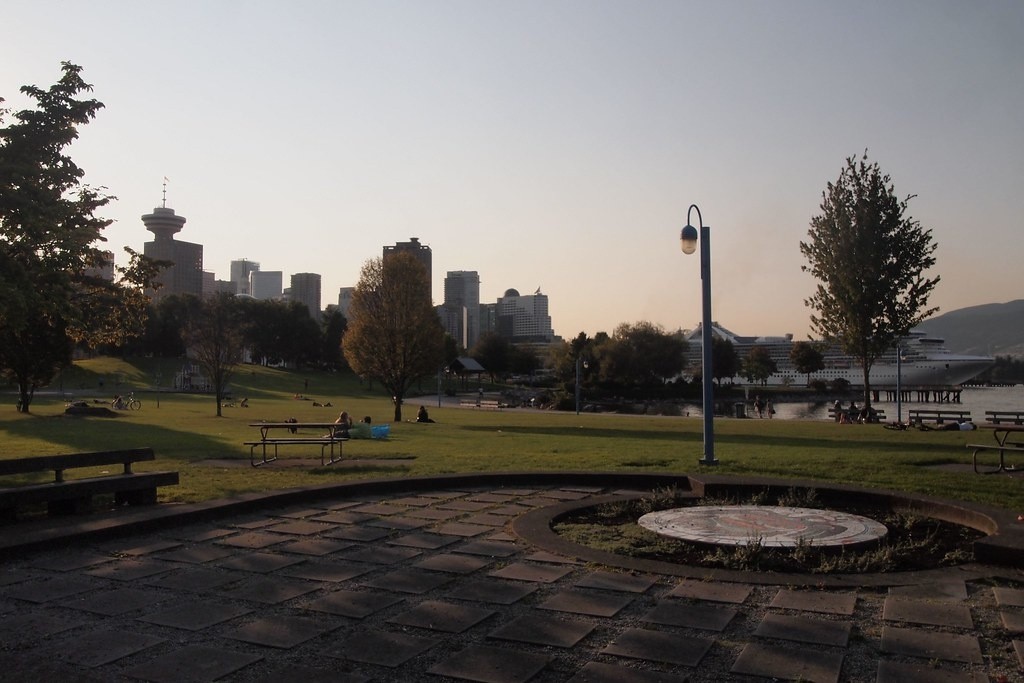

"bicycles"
[113, 392, 141, 411]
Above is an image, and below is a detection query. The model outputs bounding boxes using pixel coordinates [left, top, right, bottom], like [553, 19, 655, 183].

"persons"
[942, 421, 977, 431]
[475, 397, 481, 408]
[294, 393, 334, 407]
[99, 376, 104, 386]
[240, 398, 248, 407]
[333, 410, 352, 438]
[417, 405, 429, 423]
[478, 386, 484, 398]
[766, 399, 773, 419]
[754, 395, 763, 419]
[111, 394, 128, 410]
[833, 400, 879, 424]
[348, 416, 372, 439]
[289, 416, 298, 434]
[304, 378, 308, 389]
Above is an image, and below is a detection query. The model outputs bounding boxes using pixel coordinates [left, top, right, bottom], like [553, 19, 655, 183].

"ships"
[663, 328, 996, 388]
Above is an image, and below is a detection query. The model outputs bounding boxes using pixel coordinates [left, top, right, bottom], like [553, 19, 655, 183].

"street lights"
[681, 204, 721, 466]
[576, 355, 589, 414]
[896, 345, 909, 424]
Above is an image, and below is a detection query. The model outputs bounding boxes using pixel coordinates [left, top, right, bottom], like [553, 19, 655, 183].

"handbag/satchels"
[370, 424, 390, 439]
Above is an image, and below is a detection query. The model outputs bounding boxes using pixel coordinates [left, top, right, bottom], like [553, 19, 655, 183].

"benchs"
[244, 438, 348, 467]
[966, 443, 1024, 474]
[0, 448, 179, 523]
[480, 400, 503, 411]
[828, 409, 886, 424]
[985, 411, 1024, 425]
[909, 410, 972, 424]
[460, 400, 480, 409]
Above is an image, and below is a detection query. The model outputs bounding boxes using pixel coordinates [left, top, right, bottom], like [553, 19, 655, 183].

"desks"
[248, 423, 345, 467]
[980, 423, 1024, 474]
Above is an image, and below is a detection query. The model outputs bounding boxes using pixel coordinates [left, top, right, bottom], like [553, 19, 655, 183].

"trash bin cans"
[734, 403, 746, 418]
